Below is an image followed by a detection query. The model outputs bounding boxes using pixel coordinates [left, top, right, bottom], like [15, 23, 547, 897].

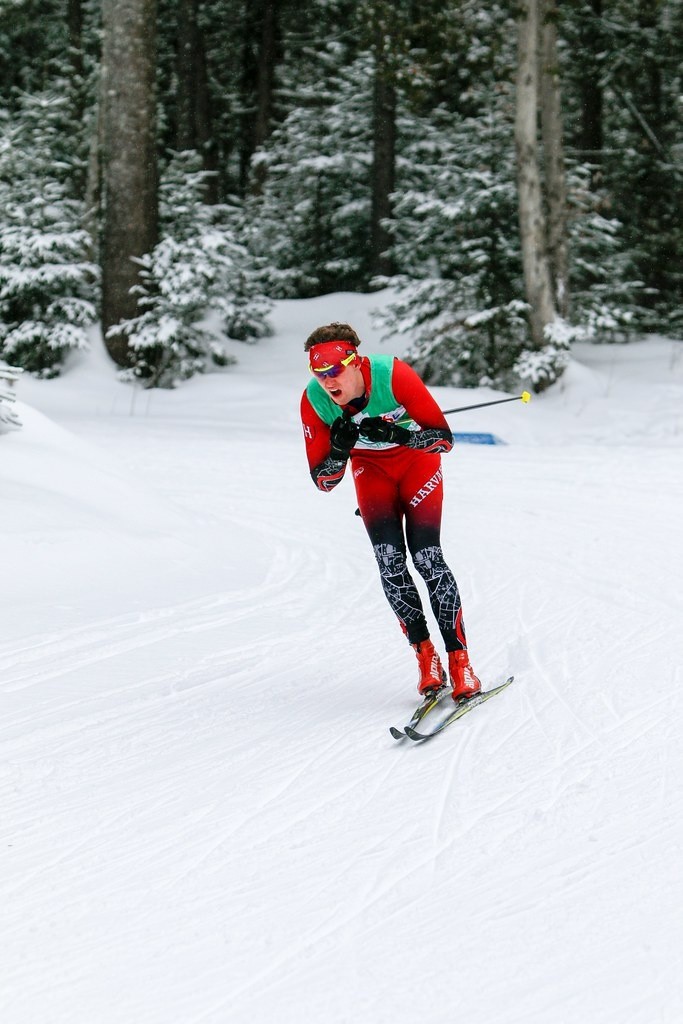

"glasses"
[313, 360, 345, 379]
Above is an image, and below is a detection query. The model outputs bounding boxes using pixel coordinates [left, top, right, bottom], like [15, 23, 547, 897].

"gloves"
[359, 416, 414, 446]
[329, 407, 359, 462]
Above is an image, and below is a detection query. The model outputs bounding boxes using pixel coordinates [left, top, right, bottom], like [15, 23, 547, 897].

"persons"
[301, 321, 481, 701]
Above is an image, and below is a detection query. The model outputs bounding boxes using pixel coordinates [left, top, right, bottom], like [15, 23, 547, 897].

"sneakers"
[411, 638, 447, 694]
[447, 650, 481, 701]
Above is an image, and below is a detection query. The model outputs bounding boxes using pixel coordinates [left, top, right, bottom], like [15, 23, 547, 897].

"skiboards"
[389, 674, 515, 743]
